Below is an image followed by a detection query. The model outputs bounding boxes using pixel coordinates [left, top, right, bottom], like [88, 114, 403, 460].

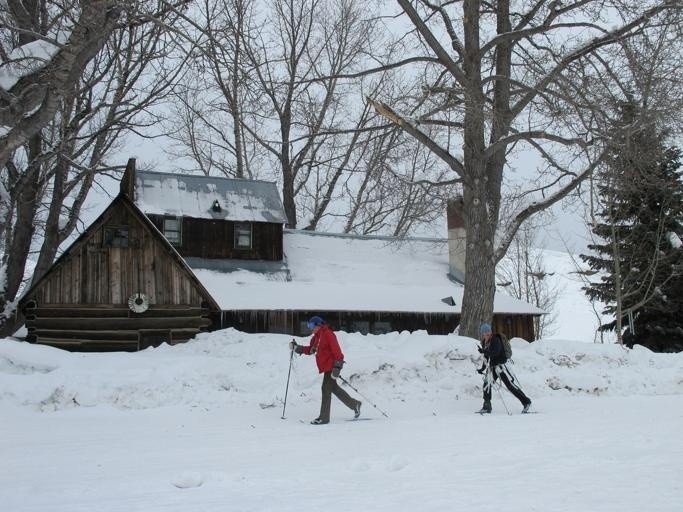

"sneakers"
[310, 418, 327, 424]
[474, 409, 490, 413]
[521, 404, 529, 413]
[353, 402, 361, 418]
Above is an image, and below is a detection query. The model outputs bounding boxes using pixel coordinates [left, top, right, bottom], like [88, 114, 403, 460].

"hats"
[309, 316, 324, 325]
[480, 324, 490, 333]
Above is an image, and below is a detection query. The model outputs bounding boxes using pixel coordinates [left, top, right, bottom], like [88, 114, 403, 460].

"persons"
[475, 323, 533, 416]
[289, 316, 363, 425]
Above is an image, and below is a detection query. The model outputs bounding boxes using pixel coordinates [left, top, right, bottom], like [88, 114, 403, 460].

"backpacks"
[498, 333, 510, 359]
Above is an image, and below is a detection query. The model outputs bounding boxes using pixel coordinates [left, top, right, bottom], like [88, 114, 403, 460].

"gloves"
[477, 368, 483, 374]
[330, 360, 344, 377]
[288, 342, 302, 354]
[478, 349, 485, 353]
